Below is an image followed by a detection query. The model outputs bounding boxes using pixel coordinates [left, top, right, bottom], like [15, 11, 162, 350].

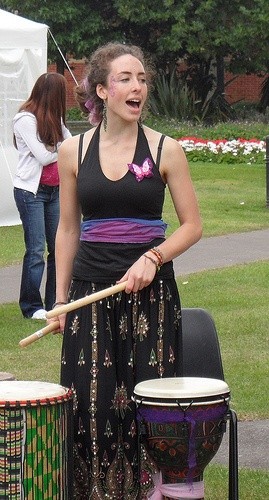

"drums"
[132, 376, 230, 500]
[0, 380, 74, 500]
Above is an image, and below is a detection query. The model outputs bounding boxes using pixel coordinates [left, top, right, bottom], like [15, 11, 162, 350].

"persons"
[12, 72, 73, 320]
[48, 41, 203, 500]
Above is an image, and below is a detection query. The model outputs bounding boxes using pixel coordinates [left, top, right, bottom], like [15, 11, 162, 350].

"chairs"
[178, 308, 239, 500]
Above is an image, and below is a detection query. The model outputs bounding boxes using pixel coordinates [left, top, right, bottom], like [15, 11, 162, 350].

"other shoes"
[32, 308, 47, 320]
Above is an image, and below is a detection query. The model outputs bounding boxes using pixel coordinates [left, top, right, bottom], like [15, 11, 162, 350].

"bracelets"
[51, 302, 68, 309]
[143, 247, 164, 272]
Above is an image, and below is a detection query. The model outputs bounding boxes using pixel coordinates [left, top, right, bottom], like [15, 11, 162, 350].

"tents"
[0, 8, 49, 226]
